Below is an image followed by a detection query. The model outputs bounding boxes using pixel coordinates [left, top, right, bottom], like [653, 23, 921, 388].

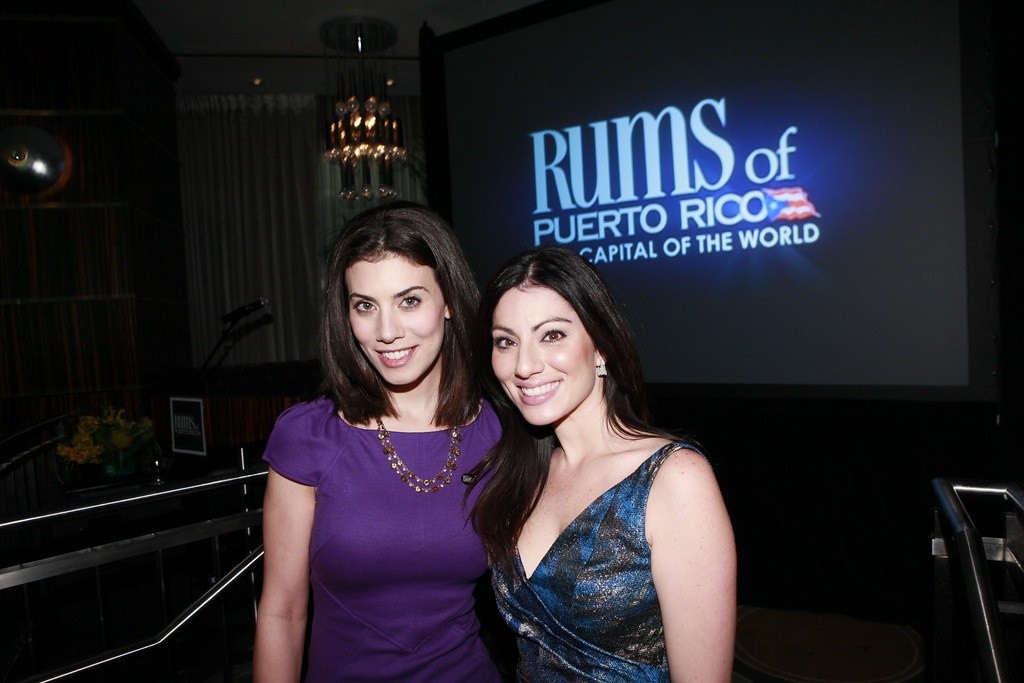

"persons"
[254, 209, 507, 683]
[462, 247, 737, 683]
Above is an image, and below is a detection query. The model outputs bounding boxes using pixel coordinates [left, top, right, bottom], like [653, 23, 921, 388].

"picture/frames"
[169, 396, 208, 457]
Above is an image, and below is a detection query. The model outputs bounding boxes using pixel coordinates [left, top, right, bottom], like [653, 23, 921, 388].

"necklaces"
[377, 417, 462, 493]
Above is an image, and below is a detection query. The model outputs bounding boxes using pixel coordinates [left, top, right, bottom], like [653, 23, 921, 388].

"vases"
[100, 456, 136, 477]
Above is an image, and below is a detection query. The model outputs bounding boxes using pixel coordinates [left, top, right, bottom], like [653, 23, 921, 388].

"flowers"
[55, 402, 155, 461]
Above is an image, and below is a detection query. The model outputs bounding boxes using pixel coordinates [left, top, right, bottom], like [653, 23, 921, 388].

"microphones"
[220, 296, 269, 325]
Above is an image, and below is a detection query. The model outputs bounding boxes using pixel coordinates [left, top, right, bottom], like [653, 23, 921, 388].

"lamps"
[313, 12, 416, 206]
[194, 298, 265, 380]
